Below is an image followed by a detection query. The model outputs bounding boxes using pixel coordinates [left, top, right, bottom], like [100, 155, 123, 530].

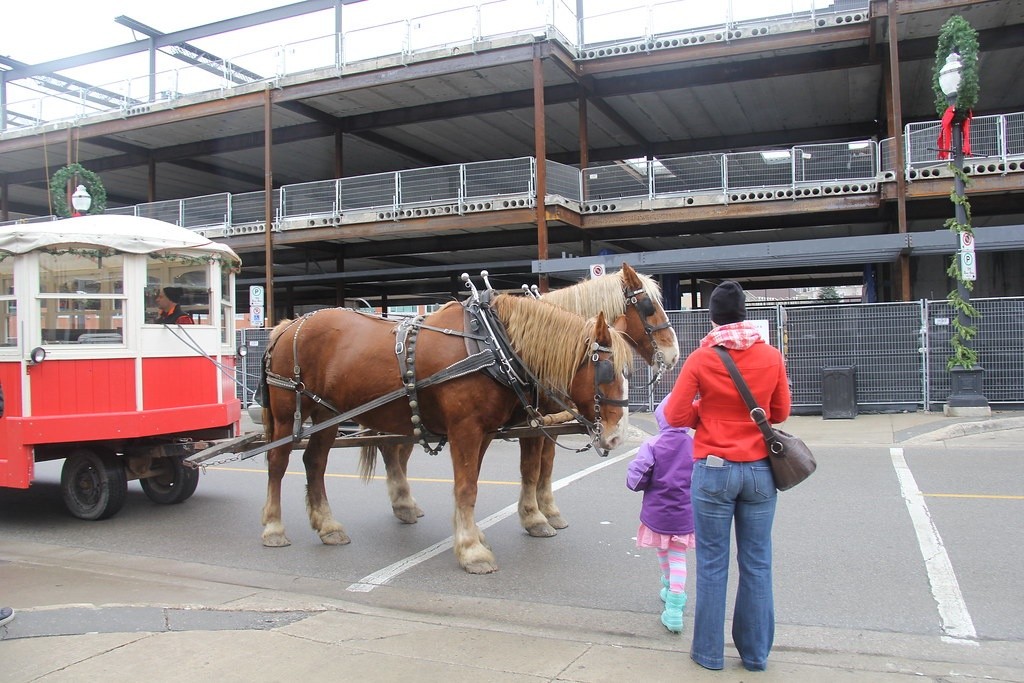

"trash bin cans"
[821, 365, 858, 419]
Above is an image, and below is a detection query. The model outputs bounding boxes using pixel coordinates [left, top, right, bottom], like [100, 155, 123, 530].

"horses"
[261, 260, 683, 577]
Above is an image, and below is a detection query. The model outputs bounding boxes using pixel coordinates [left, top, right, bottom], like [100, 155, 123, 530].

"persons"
[155, 286, 195, 325]
[663, 279, 791, 672]
[626, 392, 694, 630]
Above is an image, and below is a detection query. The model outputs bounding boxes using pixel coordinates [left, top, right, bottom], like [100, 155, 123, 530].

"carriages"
[0, 214, 681, 572]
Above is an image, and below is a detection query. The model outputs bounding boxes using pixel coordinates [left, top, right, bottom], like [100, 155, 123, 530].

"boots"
[660, 575, 687, 632]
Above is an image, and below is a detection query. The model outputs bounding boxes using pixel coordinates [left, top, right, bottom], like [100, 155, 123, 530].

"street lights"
[938, 52, 991, 418]
[72, 186, 92, 335]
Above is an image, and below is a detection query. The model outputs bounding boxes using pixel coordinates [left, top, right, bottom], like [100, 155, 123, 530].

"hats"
[709, 281, 746, 326]
[163, 287, 184, 304]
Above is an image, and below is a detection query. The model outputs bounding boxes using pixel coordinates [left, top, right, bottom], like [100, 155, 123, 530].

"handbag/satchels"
[764, 428, 817, 492]
